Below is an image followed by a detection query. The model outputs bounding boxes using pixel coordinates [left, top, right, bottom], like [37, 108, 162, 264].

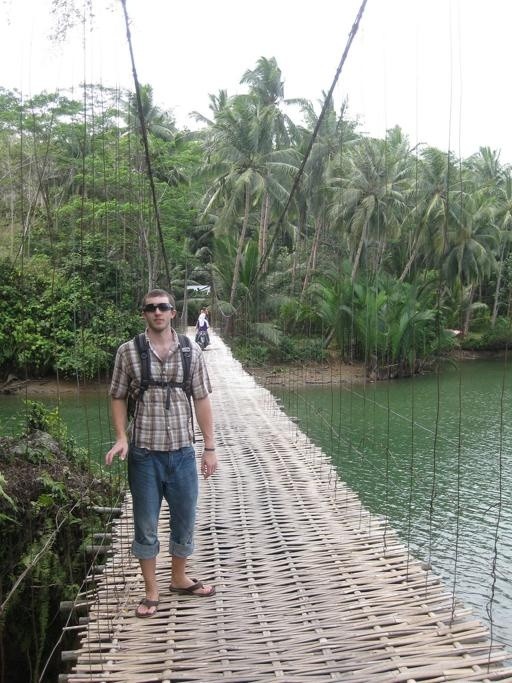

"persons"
[195, 313, 209, 342]
[104, 288, 217, 618]
[200, 306, 208, 318]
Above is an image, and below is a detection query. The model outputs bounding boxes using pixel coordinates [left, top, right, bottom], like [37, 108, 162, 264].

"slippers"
[135, 598, 159, 618]
[169, 578, 215, 596]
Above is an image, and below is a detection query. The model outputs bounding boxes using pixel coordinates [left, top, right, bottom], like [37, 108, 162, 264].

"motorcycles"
[197, 328, 209, 351]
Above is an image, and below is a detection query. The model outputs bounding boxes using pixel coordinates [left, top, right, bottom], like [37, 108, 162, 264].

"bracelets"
[204, 447, 216, 452]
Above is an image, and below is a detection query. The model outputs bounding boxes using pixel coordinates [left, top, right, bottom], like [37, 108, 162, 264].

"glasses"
[143, 302, 173, 311]
